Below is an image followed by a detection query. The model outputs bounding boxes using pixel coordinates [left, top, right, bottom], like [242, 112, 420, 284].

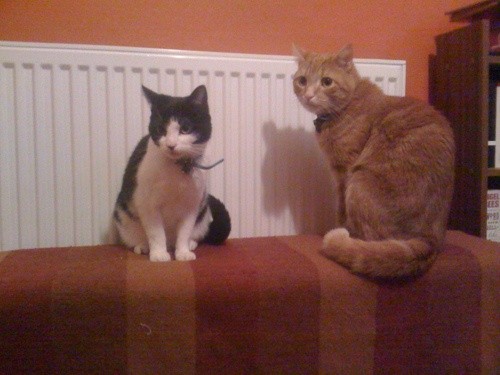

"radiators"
[0, 39, 407, 252]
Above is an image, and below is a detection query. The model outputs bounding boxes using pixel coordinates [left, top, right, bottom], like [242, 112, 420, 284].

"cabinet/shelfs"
[432, 17, 500, 243]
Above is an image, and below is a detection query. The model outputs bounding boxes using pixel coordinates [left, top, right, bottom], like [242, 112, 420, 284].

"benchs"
[0, 228, 500, 375]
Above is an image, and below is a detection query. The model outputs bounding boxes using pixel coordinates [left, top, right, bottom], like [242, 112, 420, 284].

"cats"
[111, 82, 231, 263]
[288, 38, 458, 283]
